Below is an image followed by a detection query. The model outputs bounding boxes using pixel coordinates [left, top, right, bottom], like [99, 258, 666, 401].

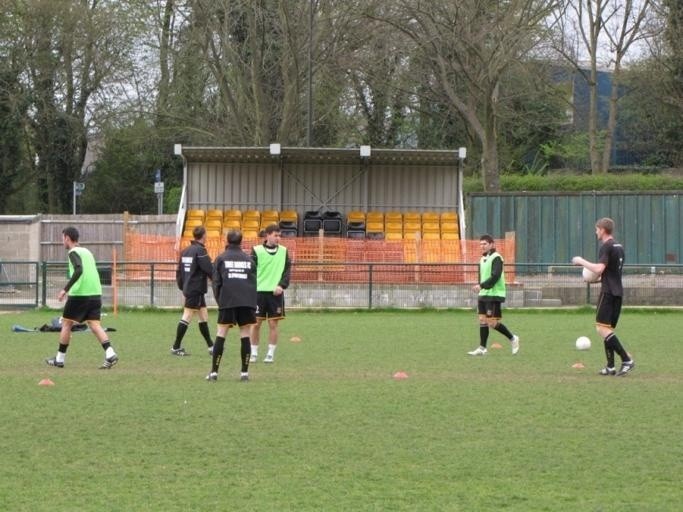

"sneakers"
[510, 334, 520, 356]
[615, 360, 636, 377]
[466, 346, 489, 357]
[96, 354, 120, 370]
[247, 352, 258, 364]
[596, 366, 615, 377]
[43, 356, 65, 368]
[262, 353, 275, 364]
[168, 348, 193, 357]
[207, 346, 213, 356]
[238, 371, 251, 382]
[203, 371, 219, 382]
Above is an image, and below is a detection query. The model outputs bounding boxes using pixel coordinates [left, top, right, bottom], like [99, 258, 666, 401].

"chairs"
[178, 209, 461, 264]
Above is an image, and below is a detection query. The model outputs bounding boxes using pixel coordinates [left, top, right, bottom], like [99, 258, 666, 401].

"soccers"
[582, 265, 600, 283]
[576, 335, 592, 351]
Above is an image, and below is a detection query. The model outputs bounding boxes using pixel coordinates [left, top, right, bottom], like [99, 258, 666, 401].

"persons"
[251, 225, 290, 363]
[170, 226, 214, 357]
[206, 230, 257, 380]
[48, 227, 119, 369]
[469, 235, 519, 356]
[571, 218, 635, 376]
